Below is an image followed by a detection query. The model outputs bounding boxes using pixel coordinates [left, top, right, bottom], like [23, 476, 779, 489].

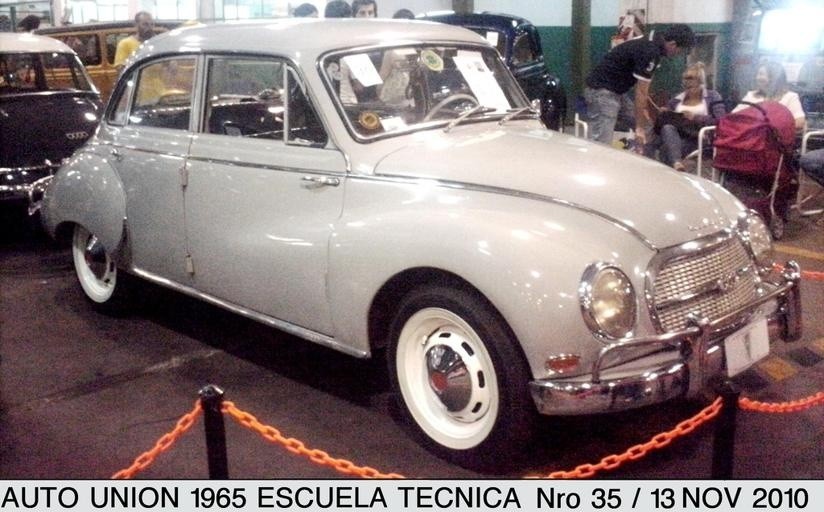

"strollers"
[687, 95, 810, 240]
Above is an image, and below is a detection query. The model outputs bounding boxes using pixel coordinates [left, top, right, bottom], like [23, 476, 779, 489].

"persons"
[346, 0, 382, 103]
[580, 21, 696, 155]
[113, 10, 177, 111]
[797, 145, 824, 187]
[325, 0, 353, 18]
[656, 61, 727, 174]
[730, 59, 807, 132]
[377, 8, 417, 108]
[293, 2, 318, 18]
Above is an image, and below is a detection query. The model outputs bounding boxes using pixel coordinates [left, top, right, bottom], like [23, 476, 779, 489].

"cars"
[31, 7, 818, 470]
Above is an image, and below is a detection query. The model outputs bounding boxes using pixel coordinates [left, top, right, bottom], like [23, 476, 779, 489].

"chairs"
[574, 90, 636, 150]
[796, 128, 824, 217]
